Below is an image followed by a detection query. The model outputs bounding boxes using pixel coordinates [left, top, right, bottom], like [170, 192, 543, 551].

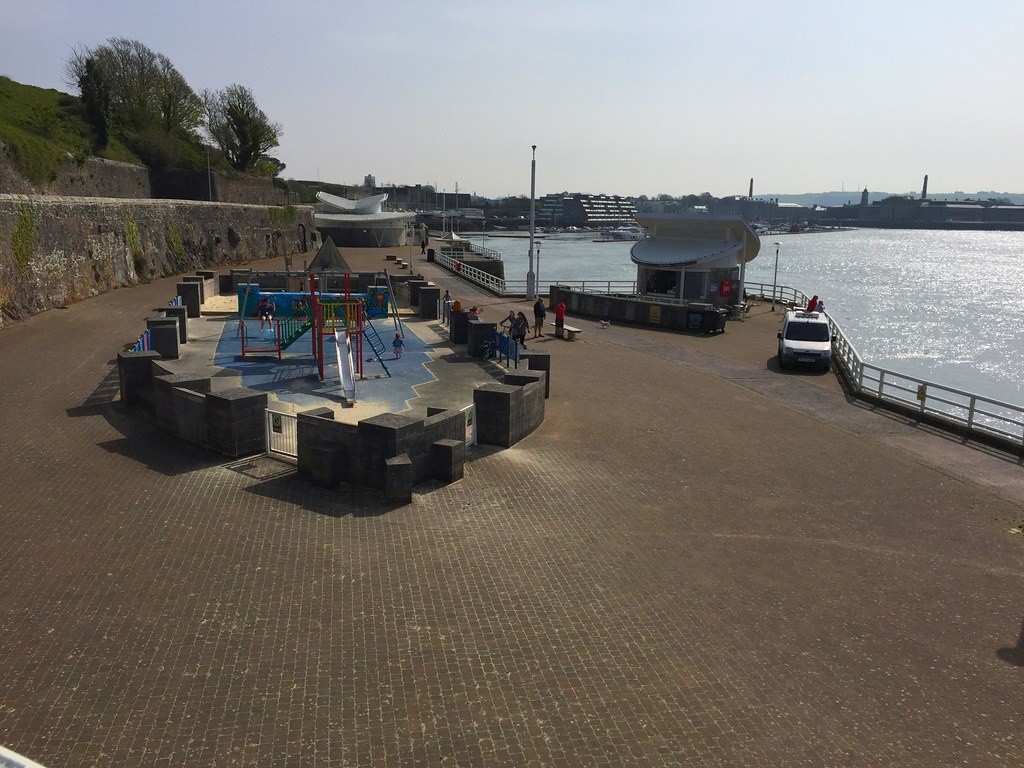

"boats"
[611, 225, 644, 240]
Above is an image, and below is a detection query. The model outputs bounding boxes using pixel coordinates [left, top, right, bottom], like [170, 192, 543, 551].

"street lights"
[534, 241, 544, 303]
[482, 221, 487, 260]
[771, 241, 783, 311]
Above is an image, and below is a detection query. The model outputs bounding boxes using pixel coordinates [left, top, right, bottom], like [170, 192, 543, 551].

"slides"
[334, 328, 358, 403]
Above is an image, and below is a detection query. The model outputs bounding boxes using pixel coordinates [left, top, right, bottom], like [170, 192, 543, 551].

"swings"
[256, 273, 274, 321]
[287, 274, 309, 321]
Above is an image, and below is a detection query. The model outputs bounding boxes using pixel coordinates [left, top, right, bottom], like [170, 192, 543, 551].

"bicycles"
[499, 323, 512, 339]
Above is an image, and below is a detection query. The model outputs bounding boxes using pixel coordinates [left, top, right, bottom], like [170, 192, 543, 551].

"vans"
[778, 308, 832, 373]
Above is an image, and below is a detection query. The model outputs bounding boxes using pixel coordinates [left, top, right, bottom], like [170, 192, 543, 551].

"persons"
[806, 295, 818, 312]
[554, 300, 566, 338]
[441, 290, 452, 302]
[743, 288, 748, 302]
[254, 299, 276, 333]
[499, 311, 519, 339]
[391, 333, 404, 359]
[814, 301, 825, 311]
[292, 300, 308, 317]
[511, 312, 530, 350]
[533, 297, 546, 338]
[420, 241, 426, 255]
